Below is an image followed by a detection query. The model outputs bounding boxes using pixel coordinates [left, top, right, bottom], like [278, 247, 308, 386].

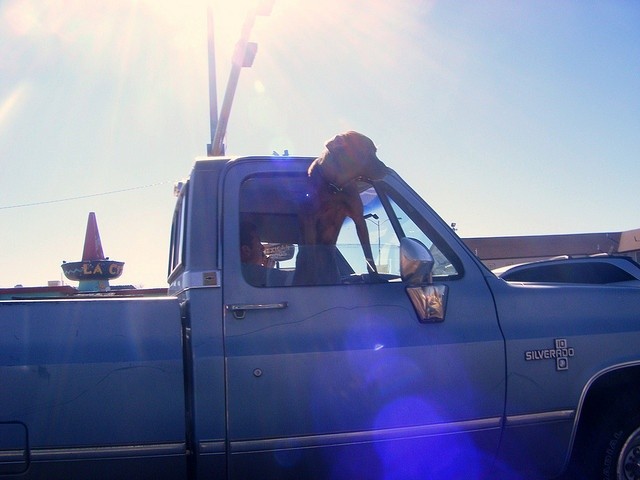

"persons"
[239, 226, 290, 287]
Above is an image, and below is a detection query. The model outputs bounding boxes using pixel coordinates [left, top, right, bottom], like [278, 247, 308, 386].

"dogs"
[291, 132, 389, 286]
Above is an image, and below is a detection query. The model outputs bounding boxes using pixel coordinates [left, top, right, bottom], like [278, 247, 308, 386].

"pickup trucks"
[2, 158, 640, 480]
